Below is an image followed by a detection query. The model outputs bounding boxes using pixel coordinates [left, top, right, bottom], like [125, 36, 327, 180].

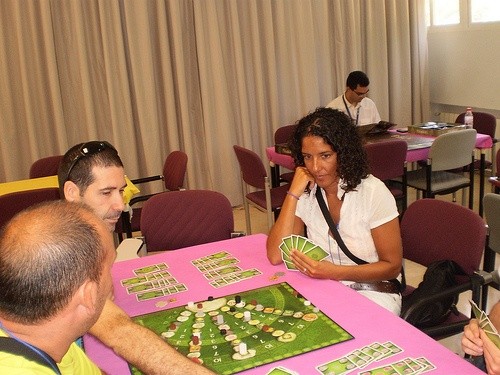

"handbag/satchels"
[399, 259, 464, 329]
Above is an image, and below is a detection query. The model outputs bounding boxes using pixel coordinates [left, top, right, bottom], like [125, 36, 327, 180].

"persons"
[0, 140, 220, 375]
[325, 71, 381, 126]
[461, 302, 500, 375]
[266, 108, 403, 317]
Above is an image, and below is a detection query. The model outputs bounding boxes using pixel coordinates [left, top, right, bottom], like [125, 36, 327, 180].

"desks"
[77, 233, 488, 375]
[266, 123, 492, 222]
[0, 173, 139, 243]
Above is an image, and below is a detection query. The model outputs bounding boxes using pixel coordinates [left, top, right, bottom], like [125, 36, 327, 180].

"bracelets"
[286, 192, 299, 200]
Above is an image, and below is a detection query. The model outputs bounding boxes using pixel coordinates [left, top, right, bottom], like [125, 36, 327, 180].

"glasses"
[63, 140, 118, 186]
[349, 87, 369, 96]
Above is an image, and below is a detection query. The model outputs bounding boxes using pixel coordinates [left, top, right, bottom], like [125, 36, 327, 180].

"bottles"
[465, 108, 473, 130]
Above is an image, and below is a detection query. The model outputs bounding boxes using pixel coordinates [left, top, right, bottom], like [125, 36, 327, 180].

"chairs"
[0, 110, 500, 345]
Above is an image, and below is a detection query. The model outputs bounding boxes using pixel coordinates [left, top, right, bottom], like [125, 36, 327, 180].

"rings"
[304, 268, 307, 272]
